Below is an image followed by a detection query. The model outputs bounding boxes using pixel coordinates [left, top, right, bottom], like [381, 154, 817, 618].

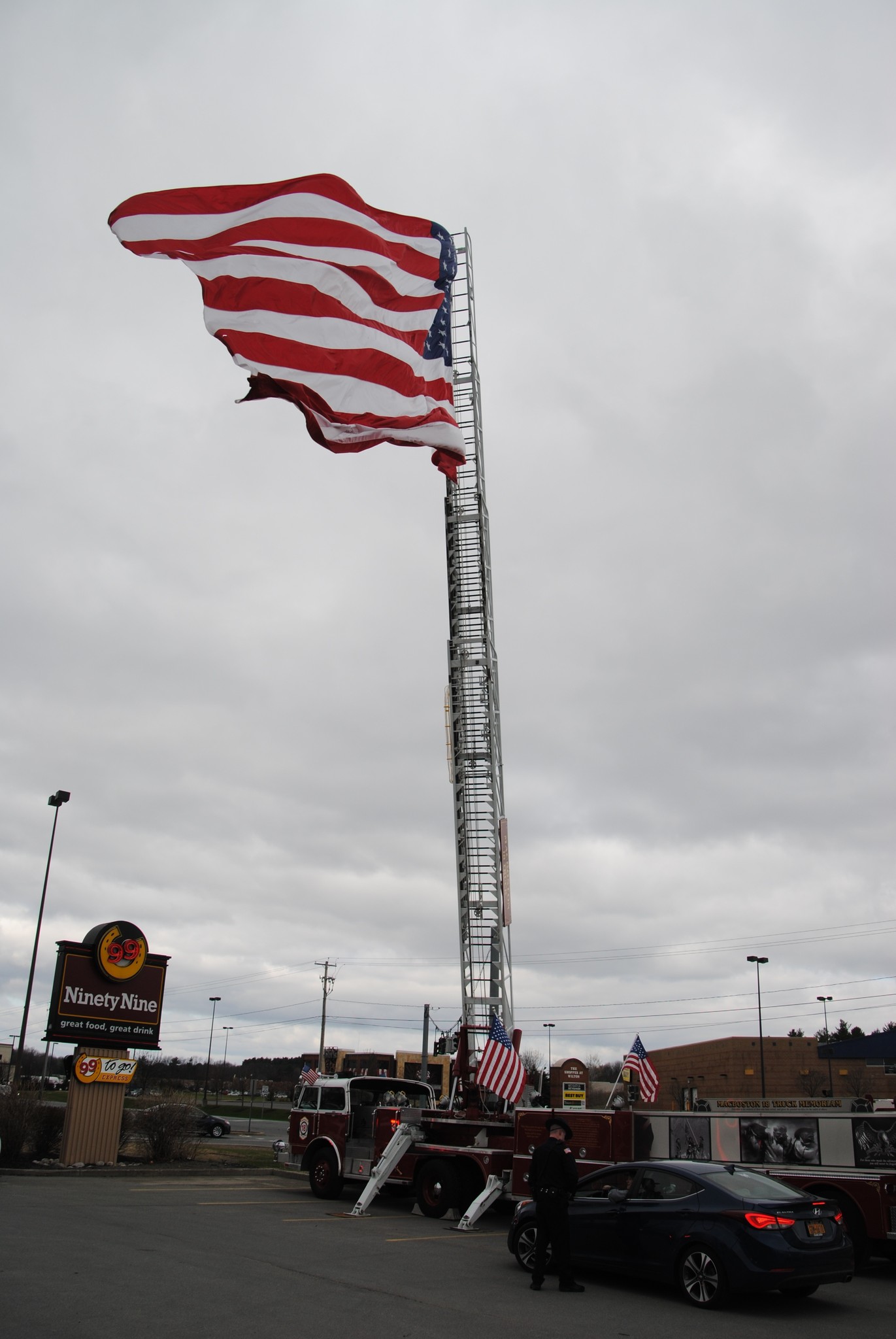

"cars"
[143, 1104, 231, 1137]
[507, 1159, 856, 1309]
[207, 1089, 248, 1096]
[260, 1092, 280, 1097]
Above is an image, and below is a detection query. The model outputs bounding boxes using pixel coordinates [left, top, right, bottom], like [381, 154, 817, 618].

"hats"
[688, 1137, 693, 1141]
[794, 1125, 817, 1140]
[699, 1136, 704, 1141]
[545, 1117, 573, 1140]
[676, 1138, 680, 1143]
[748, 1118, 771, 1128]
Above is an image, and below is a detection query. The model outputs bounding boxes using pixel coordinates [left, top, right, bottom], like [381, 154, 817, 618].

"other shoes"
[559, 1279, 585, 1292]
[529, 1282, 542, 1291]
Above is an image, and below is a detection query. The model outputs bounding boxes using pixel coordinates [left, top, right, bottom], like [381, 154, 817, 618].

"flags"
[107, 173, 466, 484]
[622, 1035, 661, 1104]
[476, 1009, 526, 1104]
[684, 1121, 690, 1146]
[301, 1065, 320, 1086]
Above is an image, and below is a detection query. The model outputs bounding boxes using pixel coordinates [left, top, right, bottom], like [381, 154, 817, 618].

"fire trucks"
[287, 223, 638, 1233]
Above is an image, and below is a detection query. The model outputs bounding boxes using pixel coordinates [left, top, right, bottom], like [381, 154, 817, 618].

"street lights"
[15, 789, 72, 1088]
[817, 996, 834, 1098]
[543, 1023, 555, 1068]
[204, 997, 221, 1098]
[6, 1035, 20, 1086]
[747, 956, 769, 1099]
[48, 1043, 58, 1089]
[221, 1026, 233, 1093]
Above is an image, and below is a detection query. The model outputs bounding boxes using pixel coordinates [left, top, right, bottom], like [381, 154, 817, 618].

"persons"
[528, 1117, 585, 1292]
[299, 1087, 336, 1104]
[675, 1136, 705, 1158]
[740, 1118, 819, 1164]
[603, 1173, 649, 1203]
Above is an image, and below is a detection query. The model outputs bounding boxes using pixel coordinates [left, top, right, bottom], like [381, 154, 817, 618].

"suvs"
[131, 1088, 143, 1097]
